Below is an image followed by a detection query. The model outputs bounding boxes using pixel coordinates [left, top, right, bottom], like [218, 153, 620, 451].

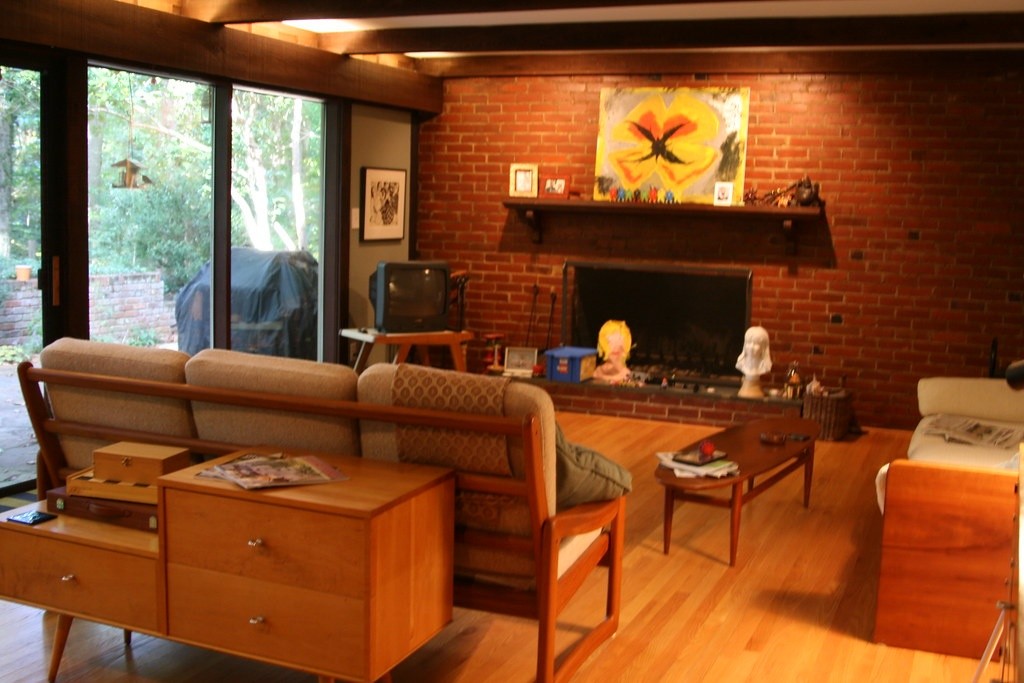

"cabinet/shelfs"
[0, 445, 455, 683]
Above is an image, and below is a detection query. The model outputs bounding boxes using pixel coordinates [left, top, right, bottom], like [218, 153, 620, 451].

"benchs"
[16, 337, 632, 682]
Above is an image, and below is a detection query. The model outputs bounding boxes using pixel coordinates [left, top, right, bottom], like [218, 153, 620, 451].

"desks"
[338, 327, 473, 374]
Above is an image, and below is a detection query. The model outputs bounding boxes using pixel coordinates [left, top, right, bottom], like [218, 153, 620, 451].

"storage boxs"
[91, 441, 190, 485]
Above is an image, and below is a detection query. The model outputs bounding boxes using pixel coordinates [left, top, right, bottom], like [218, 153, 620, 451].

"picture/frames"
[360, 166, 408, 241]
[503, 348, 538, 374]
[509, 163, 537, 200]
[538, 172, 572, 199]
[713, 181, 733, 206]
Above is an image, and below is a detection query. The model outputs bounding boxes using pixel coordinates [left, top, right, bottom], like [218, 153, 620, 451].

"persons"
[546, 178, 563, 193]
[735, 326, 773, 376]
[591, 320, 633, 386]
[369, 181, 400, 226]
[718, 188, 728, 201]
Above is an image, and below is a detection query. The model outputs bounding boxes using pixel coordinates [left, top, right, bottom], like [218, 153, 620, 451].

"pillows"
[554, 419, 632, 506]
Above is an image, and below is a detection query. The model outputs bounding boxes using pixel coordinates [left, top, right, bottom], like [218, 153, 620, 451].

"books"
[197, 453, 348, 488]
[655, 451, 740, 478]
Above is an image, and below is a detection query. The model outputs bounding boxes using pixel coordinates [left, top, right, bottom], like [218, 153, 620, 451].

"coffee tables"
[655, 418, 820, 566]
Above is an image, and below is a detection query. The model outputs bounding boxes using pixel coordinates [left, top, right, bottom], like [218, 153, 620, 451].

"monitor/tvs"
[571, 266, 753, 385]
[367, 261, 449, 333]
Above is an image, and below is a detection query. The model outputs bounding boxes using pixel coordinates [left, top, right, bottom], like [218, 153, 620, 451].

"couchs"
[873, 377, 1024, 663]
